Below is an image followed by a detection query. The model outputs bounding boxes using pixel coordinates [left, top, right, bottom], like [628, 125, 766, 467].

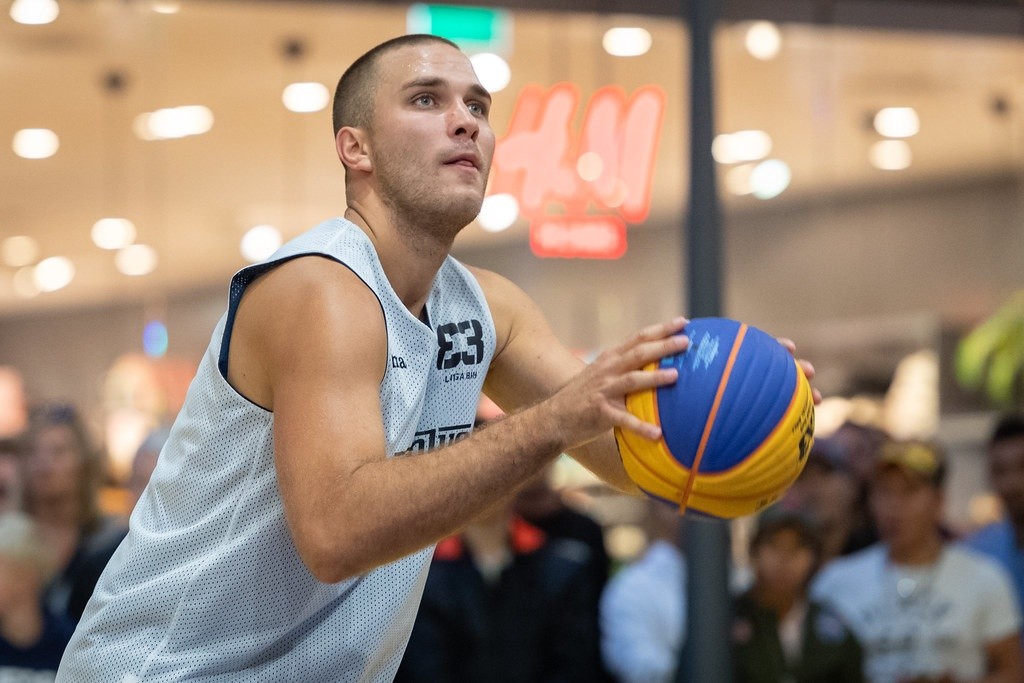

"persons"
[0, 34, 1024, 683]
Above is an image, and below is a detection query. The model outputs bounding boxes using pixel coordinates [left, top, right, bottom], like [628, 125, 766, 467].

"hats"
[809, 437, 873, 509]
[874, 440, 944, 485]
[757, 500, 821, 548]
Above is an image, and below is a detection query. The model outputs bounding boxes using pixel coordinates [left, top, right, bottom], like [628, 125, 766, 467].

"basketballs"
[613, 318, 815, 522]
